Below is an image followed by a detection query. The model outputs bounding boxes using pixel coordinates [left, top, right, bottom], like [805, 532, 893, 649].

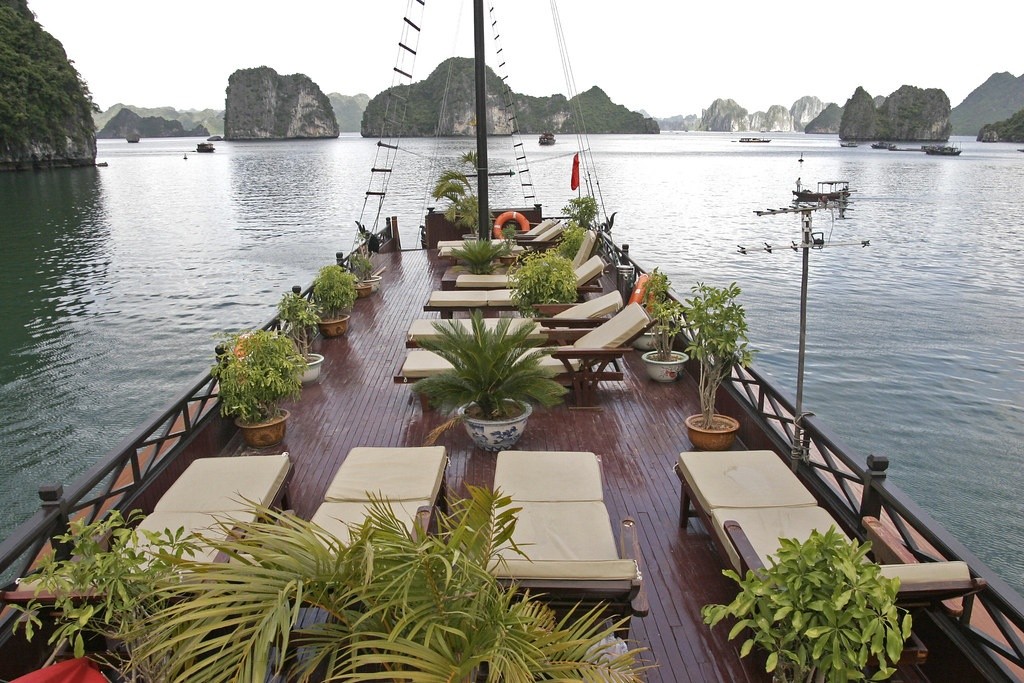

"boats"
[538, 129, 556, 146]
[197, 143, 215, 153]
[792, 189, 851, 202]
[0, 0, 1024, 682]
[125, 129, 141, 143]
[871, 142, 896, 149]
[840, 143, 857, 147]
[739, 137, 771, 143]
[888, 144, 963, 156]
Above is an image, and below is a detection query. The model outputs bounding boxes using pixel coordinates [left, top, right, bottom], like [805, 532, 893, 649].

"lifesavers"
[626, 273, 657, 314]
[493, 211, 530, 239]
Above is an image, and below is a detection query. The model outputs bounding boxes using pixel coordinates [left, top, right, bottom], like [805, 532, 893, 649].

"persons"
[796, 178, 802, 193]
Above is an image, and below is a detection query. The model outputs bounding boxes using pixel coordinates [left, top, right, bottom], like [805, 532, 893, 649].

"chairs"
[484, 448, 648, 643]
[86, 453, 295, 607]
[306, 444, 450, 559]
[395, 219, 658, 397]
[673, 450, 985, 639]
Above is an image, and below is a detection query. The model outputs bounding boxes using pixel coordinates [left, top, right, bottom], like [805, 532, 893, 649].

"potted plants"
[212, 329, 308, 451]
[311, 266, 359, 338]
[407, 309, 569, 454]
[433, 153, 492, 242]
[350, 253, 372, 298]
[639, 301, 689, 382]
[360, 241, 381, 293]
[677, 282, 759, 450]
[276, 294, 325, 387]
[630, 269, 671, 351]
